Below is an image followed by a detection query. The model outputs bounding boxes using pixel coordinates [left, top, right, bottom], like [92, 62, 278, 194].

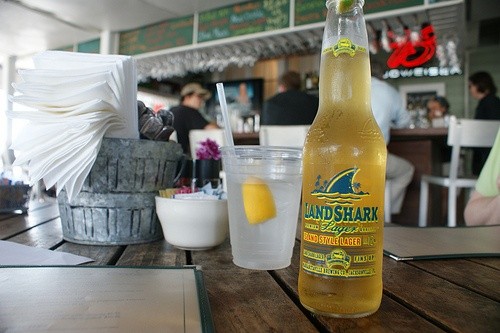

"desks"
[0, 199, 500, 333]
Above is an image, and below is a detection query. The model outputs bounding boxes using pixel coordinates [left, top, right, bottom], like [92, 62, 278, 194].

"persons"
[370, 59, 416, 215]
[468, 70, 500, 177]
[261, 70, 319, 125]
[427, 96, 467, 224]
[168, 82, 220, 157]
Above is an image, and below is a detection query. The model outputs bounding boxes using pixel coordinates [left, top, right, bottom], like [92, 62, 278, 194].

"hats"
[180, 83, 211, 99]
[435, 96, 449, 108]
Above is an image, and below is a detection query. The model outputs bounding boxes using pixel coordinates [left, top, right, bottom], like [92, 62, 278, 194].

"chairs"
[259, 125, 390, 223]
[419, 115, 500, 227]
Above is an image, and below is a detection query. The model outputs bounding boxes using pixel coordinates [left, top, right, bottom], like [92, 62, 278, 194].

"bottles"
[297, 0, 388, 319]
[408, 111, 445, 129]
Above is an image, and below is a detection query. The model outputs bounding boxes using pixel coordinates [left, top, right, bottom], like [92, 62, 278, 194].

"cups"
[218, 145, 303, 271]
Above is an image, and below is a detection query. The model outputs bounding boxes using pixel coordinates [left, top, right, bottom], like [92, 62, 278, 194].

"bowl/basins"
[155, 196, 229, 251]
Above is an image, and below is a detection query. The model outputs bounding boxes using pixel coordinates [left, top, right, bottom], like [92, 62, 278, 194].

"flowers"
[193, 137, 221, 192]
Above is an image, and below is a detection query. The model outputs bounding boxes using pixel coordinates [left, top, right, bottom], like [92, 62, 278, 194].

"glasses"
[468, 83, 474, 89]
